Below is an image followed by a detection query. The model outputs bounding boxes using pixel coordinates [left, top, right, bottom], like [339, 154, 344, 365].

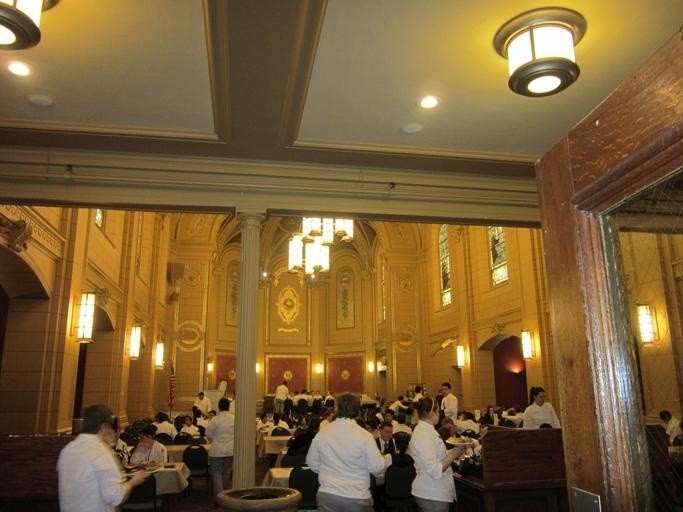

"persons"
[58, 403, 178, 509]
[473, 386, 560, 428]
[406, 383, 475, 509]
[306, 393, 410, 510]
[659, 410, 680, 445]
[178, 391, 236, 505]
[288, 389, 335, 409]
[254, 383, 290, 451]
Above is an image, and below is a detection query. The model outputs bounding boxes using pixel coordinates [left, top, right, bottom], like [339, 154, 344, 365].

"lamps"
[635, 302, 659, 349]
[457, 342, 468, 368]
[287, 218, 358, 278]
[0, 0, 66, 51]
[520, 328, 536, 360]
[493, 7, 590, 98]
[69, 287, 169, 375]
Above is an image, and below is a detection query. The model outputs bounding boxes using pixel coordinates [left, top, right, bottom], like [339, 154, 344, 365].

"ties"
[384, 441, 388, 454]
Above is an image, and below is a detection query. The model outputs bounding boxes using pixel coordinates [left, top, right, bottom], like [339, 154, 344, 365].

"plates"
[123, 467, 163, 476]
[445, 438, 477, 446]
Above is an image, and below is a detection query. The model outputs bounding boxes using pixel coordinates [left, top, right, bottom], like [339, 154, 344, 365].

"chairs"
[61, 394, 571, 512]
[643, 418, 682, 511]
